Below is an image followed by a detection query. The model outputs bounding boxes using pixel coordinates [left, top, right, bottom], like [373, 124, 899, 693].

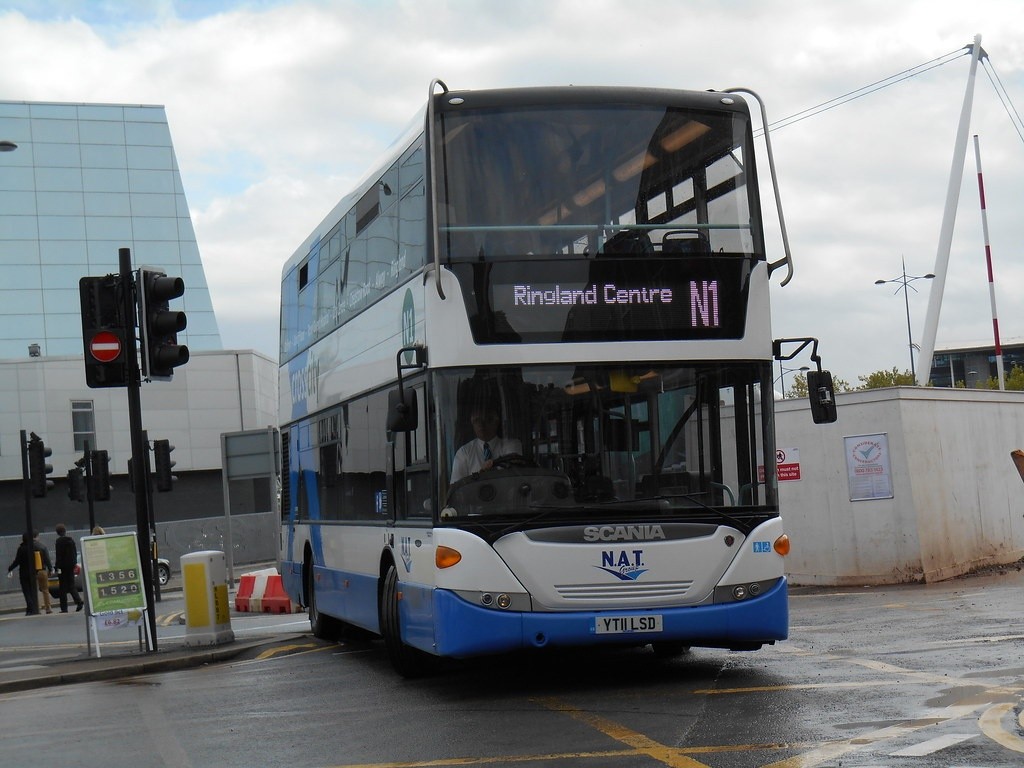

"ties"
[483, 442, 492, 462]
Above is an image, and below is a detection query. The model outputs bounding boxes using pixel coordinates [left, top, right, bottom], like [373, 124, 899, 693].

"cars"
[48, 546, 172, 599]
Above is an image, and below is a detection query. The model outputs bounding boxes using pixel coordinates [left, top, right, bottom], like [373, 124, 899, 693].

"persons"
[8, 533, 32, 616]
[54, 524, 84, 614]
[447, 407, 523, 513]
[91, 526, 104, 536]
[32, 529, 53, 614]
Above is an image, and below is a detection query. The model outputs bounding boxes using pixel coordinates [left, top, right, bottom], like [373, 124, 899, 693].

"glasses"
[472, 415, 499, 423]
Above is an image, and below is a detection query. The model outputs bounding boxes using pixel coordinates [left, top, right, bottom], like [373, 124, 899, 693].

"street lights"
[874, 253, 936, 385]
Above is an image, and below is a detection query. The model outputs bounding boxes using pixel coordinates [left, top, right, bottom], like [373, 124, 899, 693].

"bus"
[276, 79, 839, 684]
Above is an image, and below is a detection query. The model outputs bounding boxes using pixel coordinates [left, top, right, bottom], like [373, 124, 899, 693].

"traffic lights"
[30, 439, 55, 500]
[155, 438, 179, 494]
[139, 267, 190, 382]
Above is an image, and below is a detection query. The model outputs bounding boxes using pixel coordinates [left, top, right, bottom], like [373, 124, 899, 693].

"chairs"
[582, 229, 712, 255]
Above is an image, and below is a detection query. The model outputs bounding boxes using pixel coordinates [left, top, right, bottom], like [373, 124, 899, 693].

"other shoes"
[25, 610, 41, 616]
[58, 609, 68, 613]
[75, 601, 84, 612]
[45, 610, 54, 615]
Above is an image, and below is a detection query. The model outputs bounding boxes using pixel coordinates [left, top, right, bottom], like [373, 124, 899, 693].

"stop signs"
[90, 331, 122, 362]
